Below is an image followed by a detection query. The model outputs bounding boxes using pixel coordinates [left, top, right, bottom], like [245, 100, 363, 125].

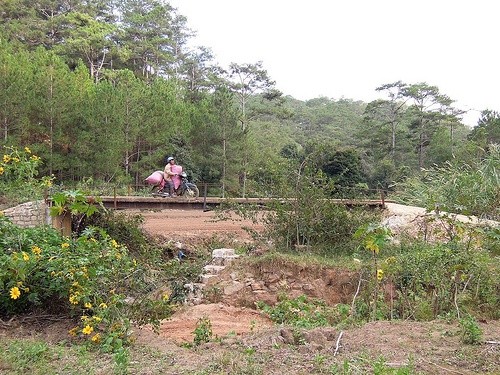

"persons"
[163, 156, 181, 198]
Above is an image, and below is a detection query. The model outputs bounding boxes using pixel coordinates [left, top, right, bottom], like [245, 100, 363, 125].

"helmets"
[167, 157, 174, 162]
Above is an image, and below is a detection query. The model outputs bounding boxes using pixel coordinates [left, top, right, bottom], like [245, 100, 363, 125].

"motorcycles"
[151, 170, 200, 200]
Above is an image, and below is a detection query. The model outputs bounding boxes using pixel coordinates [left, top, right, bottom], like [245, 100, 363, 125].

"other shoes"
[170, 194, 177, 198]
